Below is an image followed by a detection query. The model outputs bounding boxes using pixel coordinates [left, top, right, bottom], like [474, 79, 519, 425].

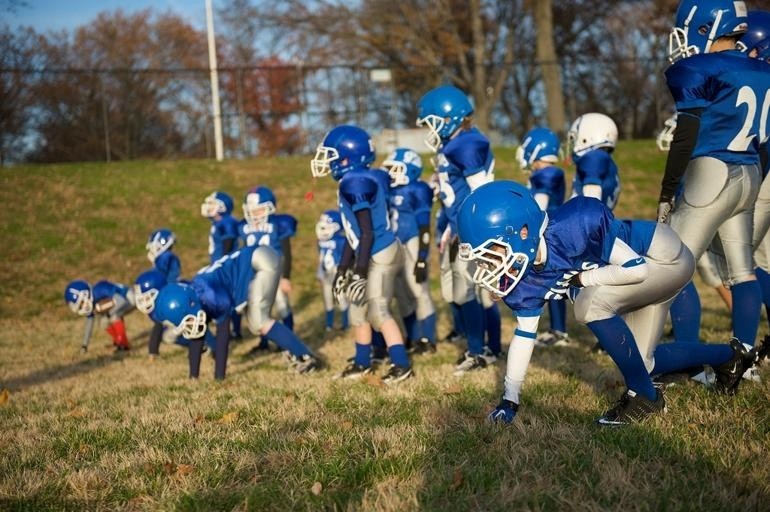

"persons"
[656, 0, 769, 385]
[417, 83, 502, 373]
[63, 230, 215, 358]
[656, 11, 769, 313]
[234, 185, 298, 352]
[515, 125, 569, 347]
[456, 182, 759, 429]
[566, 111, 620, 208]
[197, 189, 238, 262]
[154, 245, 320, 380]
[309, 124, 451, 385]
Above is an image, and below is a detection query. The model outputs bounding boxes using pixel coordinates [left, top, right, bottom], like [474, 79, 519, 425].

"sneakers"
[296, 339, 496, 383]
[538, 329, 763, 426]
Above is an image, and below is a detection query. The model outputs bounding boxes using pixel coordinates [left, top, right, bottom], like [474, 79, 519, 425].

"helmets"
[669, 1, 770, 64]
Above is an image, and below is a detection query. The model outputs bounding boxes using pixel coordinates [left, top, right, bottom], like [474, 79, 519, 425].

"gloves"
[487, 400, 519, 426]
[658, 201, 672, 225]
